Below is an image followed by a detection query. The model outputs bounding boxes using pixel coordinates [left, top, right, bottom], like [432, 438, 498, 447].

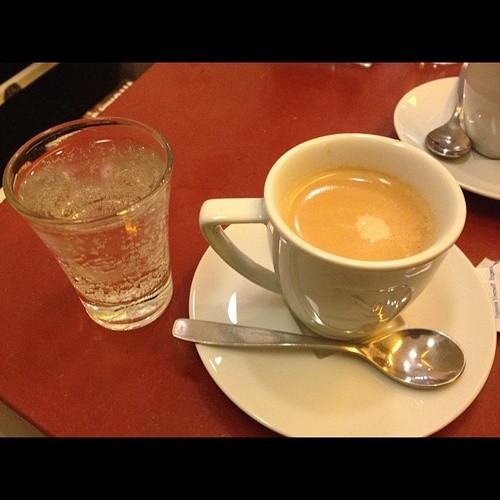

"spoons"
[171, 318, 466, 390]
[424, 63, 471, 159]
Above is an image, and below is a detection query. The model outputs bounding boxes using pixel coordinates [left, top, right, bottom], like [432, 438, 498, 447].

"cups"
[198, 133, 467, 344]
[463, 63, 500, 160]
[2, 116, 175, 331]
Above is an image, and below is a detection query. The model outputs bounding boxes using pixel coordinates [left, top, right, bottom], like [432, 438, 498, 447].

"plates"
[393, 76, 500, 201]
[189, 224, 497, 437]
